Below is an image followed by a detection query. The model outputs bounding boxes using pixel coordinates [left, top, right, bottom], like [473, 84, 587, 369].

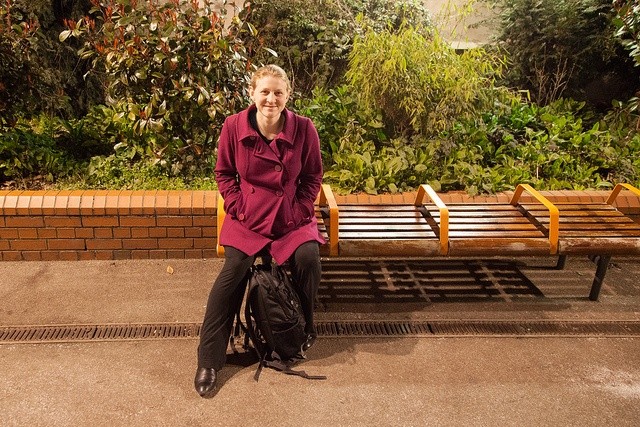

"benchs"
[216, 183, 640, 301]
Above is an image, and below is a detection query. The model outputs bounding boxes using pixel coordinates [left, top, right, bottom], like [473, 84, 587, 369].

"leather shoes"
[305, 333, 316, 351]
[193, 367, 218, 397]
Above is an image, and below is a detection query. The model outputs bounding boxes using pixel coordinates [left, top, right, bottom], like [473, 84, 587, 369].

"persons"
[194, 64, 327, 395]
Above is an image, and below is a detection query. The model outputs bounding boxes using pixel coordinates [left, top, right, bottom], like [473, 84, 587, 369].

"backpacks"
[230, 257, 328, 382]
